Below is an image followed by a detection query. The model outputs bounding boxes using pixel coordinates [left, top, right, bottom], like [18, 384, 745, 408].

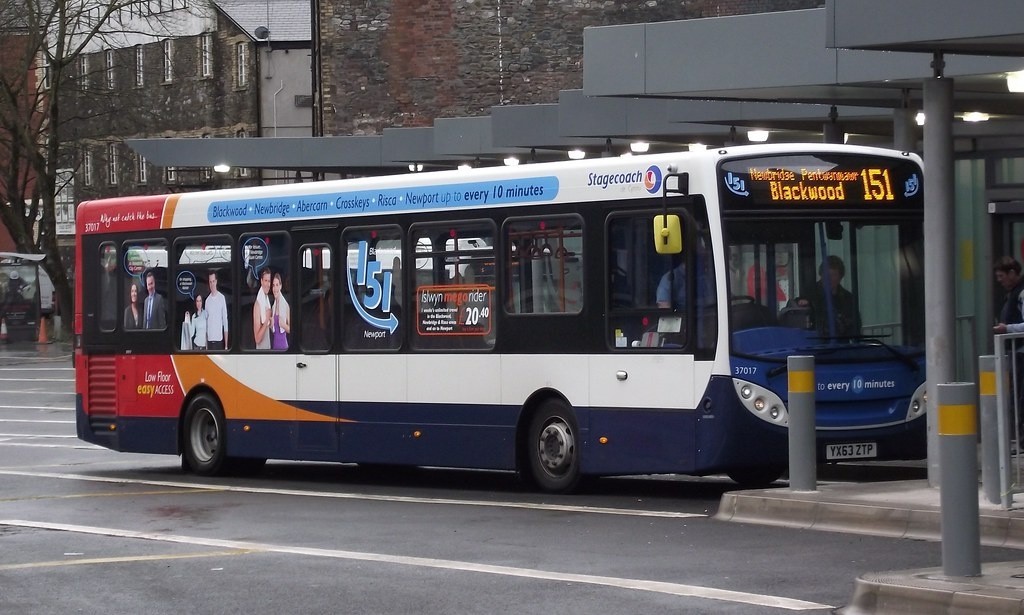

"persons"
[142, 271, 166, 328]
[184, 291, 206, 349]
[124, 283, 143, 328]
[253, 266, 273, 349]
[2, 271, 24, 303]
[656, 231, 717, 349]
[992, 255, 1024, 454]
[788, 256, 863, 342]
[270, 272, 290, 350]
[205, 269, 228, 350]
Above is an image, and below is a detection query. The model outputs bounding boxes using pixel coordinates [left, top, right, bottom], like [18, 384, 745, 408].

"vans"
[38, 265, 55, 314]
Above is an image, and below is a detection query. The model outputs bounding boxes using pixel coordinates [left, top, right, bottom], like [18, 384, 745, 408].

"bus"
[73, 143, 925, 492]
[130, 236, 521, 349]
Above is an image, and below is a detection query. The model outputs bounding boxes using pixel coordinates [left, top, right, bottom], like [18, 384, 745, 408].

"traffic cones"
[36, 317, 52, 345]
[0, 319, 11, 344]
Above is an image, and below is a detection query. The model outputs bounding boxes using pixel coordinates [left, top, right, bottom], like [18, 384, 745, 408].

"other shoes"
[1011, 447, 1024, 454]
